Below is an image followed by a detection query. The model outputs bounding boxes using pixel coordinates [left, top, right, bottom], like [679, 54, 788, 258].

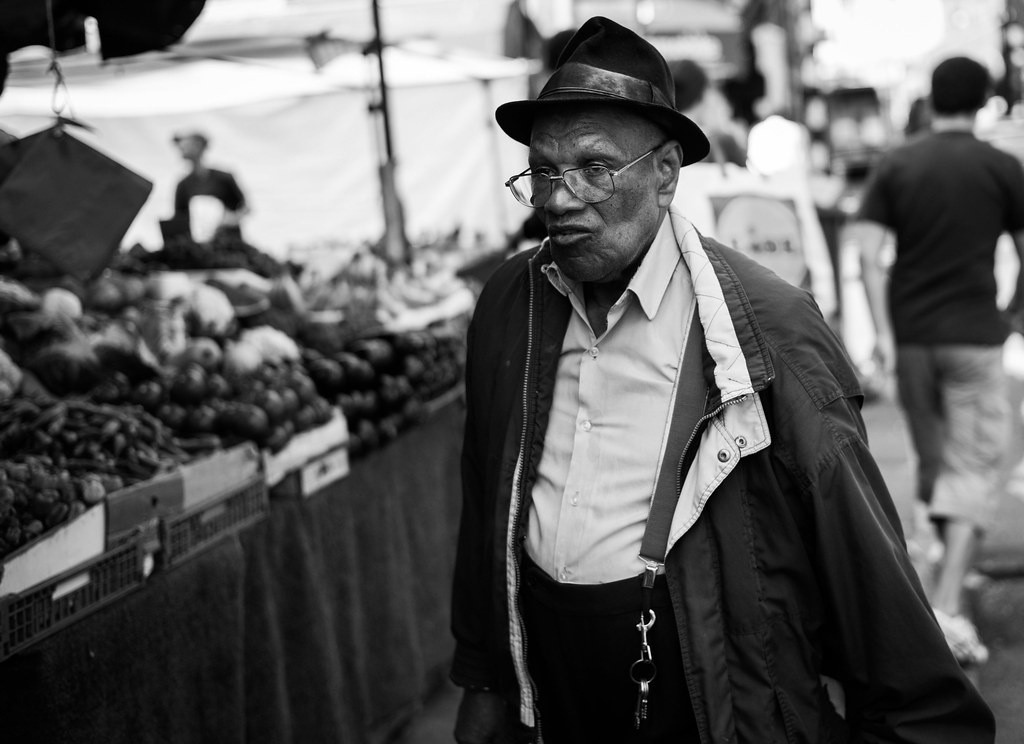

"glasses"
[505, 144, 661, 208]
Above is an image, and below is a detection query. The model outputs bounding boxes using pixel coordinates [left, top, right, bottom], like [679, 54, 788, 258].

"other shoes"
[934, 606, 990, 664]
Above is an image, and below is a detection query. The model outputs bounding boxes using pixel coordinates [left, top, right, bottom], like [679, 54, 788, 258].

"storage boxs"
[161, 474, 267, 566]
[1, 524, 147, 663]
[269, 435, 364, 519]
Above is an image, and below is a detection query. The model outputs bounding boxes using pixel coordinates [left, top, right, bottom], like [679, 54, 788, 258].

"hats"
[495, 15, 711, 167]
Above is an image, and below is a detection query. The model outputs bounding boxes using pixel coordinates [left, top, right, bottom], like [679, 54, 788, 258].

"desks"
[0, 389, 467, 744]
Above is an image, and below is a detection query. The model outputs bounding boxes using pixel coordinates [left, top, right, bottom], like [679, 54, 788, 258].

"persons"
[853, 63, 1024, 663]
[167, 125, 250, 245]
[448, 15, 996, 744]
[671, 23, 1024, 369]
